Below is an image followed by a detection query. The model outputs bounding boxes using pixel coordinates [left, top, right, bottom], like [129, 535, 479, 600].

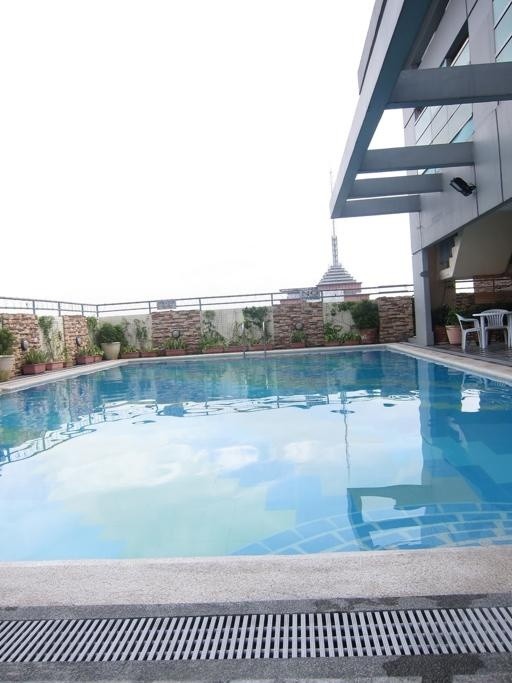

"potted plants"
[0, 328, 16, 382]
[324, 328, 361, 346]
[98, 323, 125, 360]
[121, 338, 188, 359]
[350, 299, 379, 343]
[290, 330, 306, 348]
[201, 332, 273, 354]
[21, 343, 105, 374]
[445, 309, 467, 344]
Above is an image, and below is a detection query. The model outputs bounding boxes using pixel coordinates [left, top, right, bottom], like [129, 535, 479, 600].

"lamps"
[449, 177, 476, 197]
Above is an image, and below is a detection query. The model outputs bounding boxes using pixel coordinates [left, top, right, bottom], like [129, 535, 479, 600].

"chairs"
[454, 309, 512, 350]
